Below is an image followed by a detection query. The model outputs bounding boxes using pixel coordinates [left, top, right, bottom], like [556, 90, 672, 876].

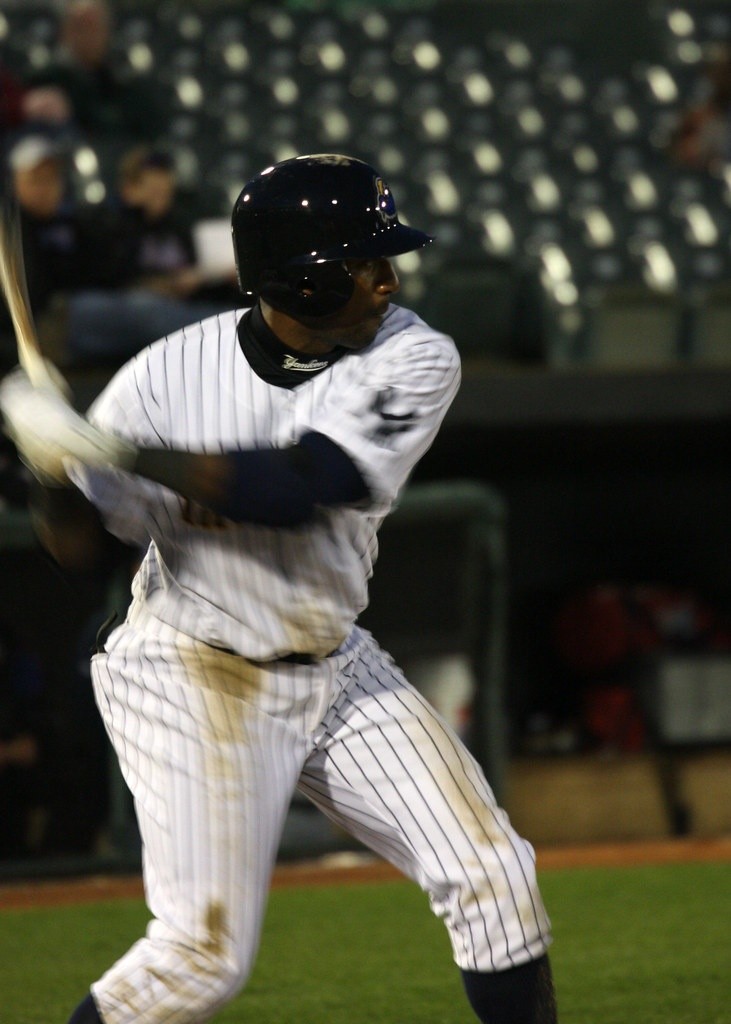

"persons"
[0, 0, 238, 361]
[0, 153, 558, 1024]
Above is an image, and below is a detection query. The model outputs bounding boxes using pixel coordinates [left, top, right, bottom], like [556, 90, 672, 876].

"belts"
[207, 647, 313, 664]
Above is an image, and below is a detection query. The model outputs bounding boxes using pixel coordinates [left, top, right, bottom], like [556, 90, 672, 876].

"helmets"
[230, 154, 433, 316]
